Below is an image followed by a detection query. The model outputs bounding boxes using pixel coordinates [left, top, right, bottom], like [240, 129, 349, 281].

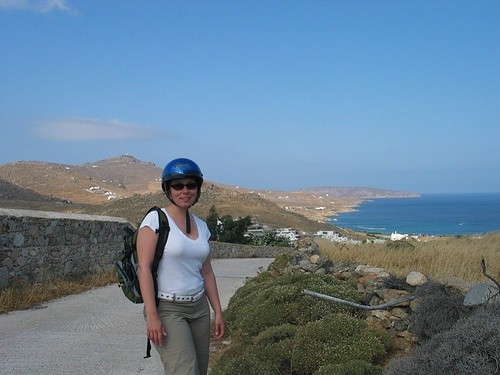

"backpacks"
[115, 206, 171, 304]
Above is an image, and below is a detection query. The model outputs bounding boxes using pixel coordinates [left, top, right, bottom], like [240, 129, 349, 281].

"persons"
[115, 158, 224, 375]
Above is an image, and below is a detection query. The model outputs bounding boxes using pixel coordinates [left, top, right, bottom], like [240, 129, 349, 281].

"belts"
[157, 287, 205, 303]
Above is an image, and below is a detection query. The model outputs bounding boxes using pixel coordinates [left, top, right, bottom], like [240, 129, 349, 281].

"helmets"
[161, 158, 204, 193]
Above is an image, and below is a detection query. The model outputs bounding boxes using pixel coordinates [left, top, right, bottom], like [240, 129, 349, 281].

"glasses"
[170, 182, 198, 190]
